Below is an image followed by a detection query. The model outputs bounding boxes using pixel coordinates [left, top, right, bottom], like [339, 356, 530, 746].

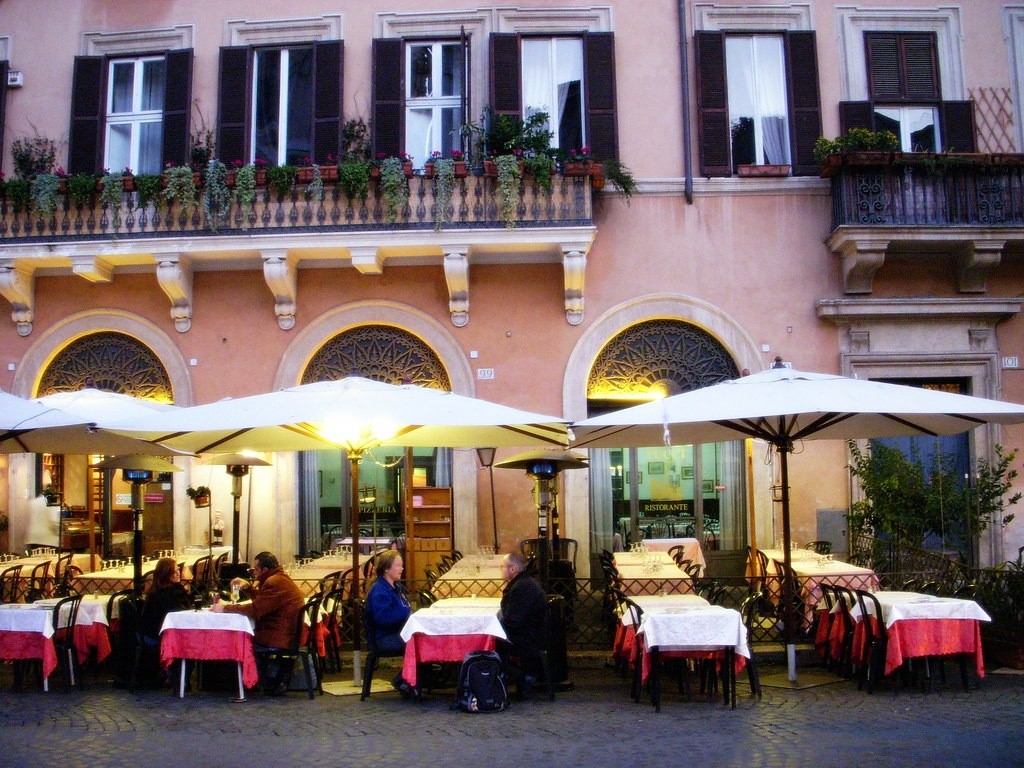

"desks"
[323, 521, 405, 549]
[400, 607, 512, 701]
[744, 548, 826, 592]
[430, 567, 512, 599]
[160, 546, 242, 578]
[612, 594, 709, 657]
[780, 562, 880, 634]
[34, 595, 129, 663]
[75, 555, 218, 595]
[284, 568, 364, 599]
[305, 554, 378, 576]
[159, 598, 344, 701]
[613, 552, 675, 564]
[0, 565, 56, 603]
[816, 591, 937, 661]
[0, 554, 103, 575]
[430, 598, 503, 608]
[0, 603, 92, 691]
[643, 537, 707, 578]
[453, 554, 506, 567]
[866, 597, 992, 692]
[621, 607, 751, 709]
[611, 565, 697, 595]
[620, 518, 720, 541]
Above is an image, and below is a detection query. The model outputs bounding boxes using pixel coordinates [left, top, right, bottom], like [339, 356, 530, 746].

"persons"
[498, 552, 555, 702]
[364, 550, 420, 702]
[139, 557, 196, 692]
[213, 552, 304, 693]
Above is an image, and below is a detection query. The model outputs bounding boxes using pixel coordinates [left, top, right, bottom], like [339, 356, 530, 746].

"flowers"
[0, 106, 643, 232]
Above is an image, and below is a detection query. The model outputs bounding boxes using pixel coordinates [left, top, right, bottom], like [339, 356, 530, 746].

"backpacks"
[452, 650, 510, 714]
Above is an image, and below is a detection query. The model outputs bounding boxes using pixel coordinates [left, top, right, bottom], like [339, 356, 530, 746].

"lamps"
[477, 447, 497, 467]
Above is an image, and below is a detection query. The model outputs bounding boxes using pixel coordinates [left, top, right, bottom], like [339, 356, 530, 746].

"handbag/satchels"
[289, 653, 321, 690]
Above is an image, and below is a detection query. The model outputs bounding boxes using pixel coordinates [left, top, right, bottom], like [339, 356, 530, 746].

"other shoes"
[391, 674, 426, 701]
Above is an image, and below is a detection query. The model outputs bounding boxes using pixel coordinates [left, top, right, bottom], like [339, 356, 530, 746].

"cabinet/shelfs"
[404, 486, 453, 593]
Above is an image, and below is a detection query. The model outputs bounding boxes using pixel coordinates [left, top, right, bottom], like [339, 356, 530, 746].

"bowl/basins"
[40, 604, 56, 609]
[68, 528, 80, 534]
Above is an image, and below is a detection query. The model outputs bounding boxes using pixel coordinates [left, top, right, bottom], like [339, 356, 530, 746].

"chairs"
[416, 587, 437, 608]
[648, 517, 719, 550]
[626, 529, 651, 549]
[328, 525, 373, 553]
[0, 538, 982, 699]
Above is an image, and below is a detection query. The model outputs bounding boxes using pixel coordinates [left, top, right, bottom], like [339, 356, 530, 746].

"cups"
[472, 594, 476, 602]
[868, 586, 877, 595]
[94, 591, 98, 599]
[70, 590, 75, 597]
[195, 595, 202, 610]
[659, 590, 667, 598]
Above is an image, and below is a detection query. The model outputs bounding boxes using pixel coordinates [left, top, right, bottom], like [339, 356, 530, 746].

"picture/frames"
[717, 480, 720, 492]
[648, 462, 664, 474]
[703, 480, 713, 493]
[682, 467, 694, 479]
[626, 471, 642, 484]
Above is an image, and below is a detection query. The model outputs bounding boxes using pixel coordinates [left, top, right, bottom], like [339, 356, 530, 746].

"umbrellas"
[88, 373, 574, 688]
[0, 389, 200, 572]
[568, 357, 1024, 681]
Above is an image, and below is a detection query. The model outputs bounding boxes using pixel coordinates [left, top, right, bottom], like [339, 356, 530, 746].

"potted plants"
[894, 143, 1024, 178]
[44, 488, 58, 504]
[448, 117, 487, 178]
[186, 486, 211, 508]
[812, 128, 901, 178]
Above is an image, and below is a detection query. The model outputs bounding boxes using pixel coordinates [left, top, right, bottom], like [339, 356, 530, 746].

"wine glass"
[231, 585, 239, 604]
[818, 554, 835, 569]
[283, 558, 312, 577]
[127, 557, 133, 569]
[630, 542, 663, 575]
[0, 554, 20, 567]
[31, 547, 56, 560]
[800, 550, 814, 562]
[776, 539, 784, 552]
[142, 556, 151, 568]
[158, 546, 209, 561]
[477, 545, 495, 560]
[790, 542, 798, 552]
[323, 546, 353, 561]
[103, 560, 126, 574]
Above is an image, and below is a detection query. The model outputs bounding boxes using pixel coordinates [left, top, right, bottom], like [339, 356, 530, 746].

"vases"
[564, 161, 593, 175]
[372, 162, 413, 178]
[162, 172, 203, 187]
[592, 163, 604, 188]
[227, 170, 267, 186]
[485, 159, 525, 177]
[425, 162, 468, 177]
[298, 166, 340, 181]
[98, 176, 134, 191]
[59, 179, 68, 194]
[737, 164, 790, 176]
